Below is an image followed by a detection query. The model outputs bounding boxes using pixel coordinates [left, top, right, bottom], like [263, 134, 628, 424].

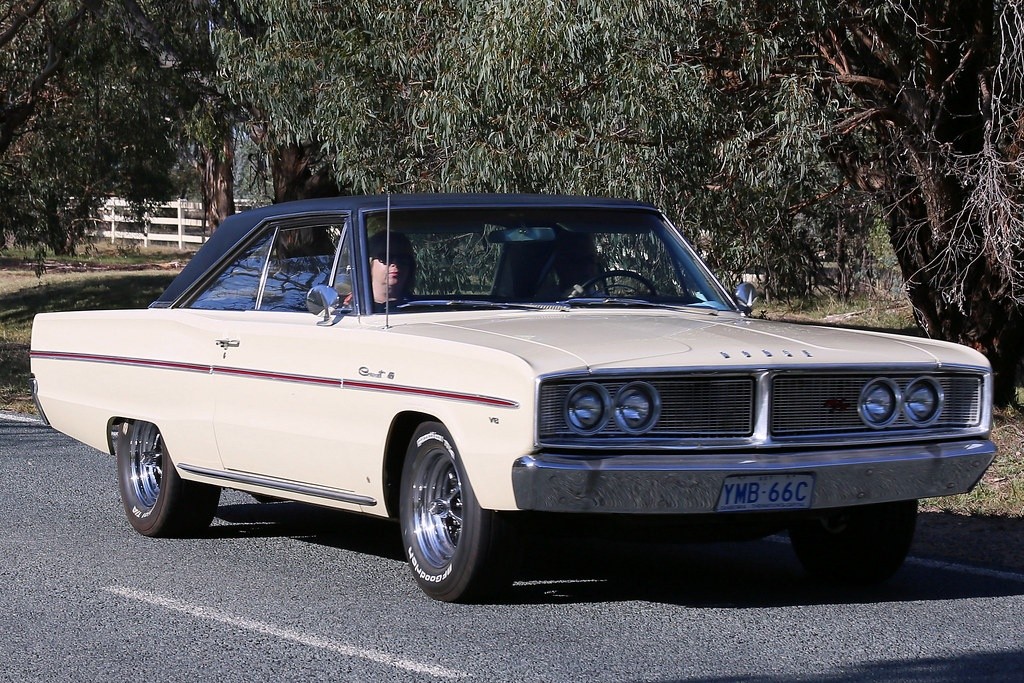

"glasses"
[369, 250, 414, 266]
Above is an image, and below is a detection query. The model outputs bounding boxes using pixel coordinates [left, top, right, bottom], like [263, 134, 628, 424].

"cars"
[28, 189, 1002, 602]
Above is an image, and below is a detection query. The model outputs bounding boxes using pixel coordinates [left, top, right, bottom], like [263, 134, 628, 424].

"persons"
[319, 231, 415, 312]
[517, 232, 610, 302]
